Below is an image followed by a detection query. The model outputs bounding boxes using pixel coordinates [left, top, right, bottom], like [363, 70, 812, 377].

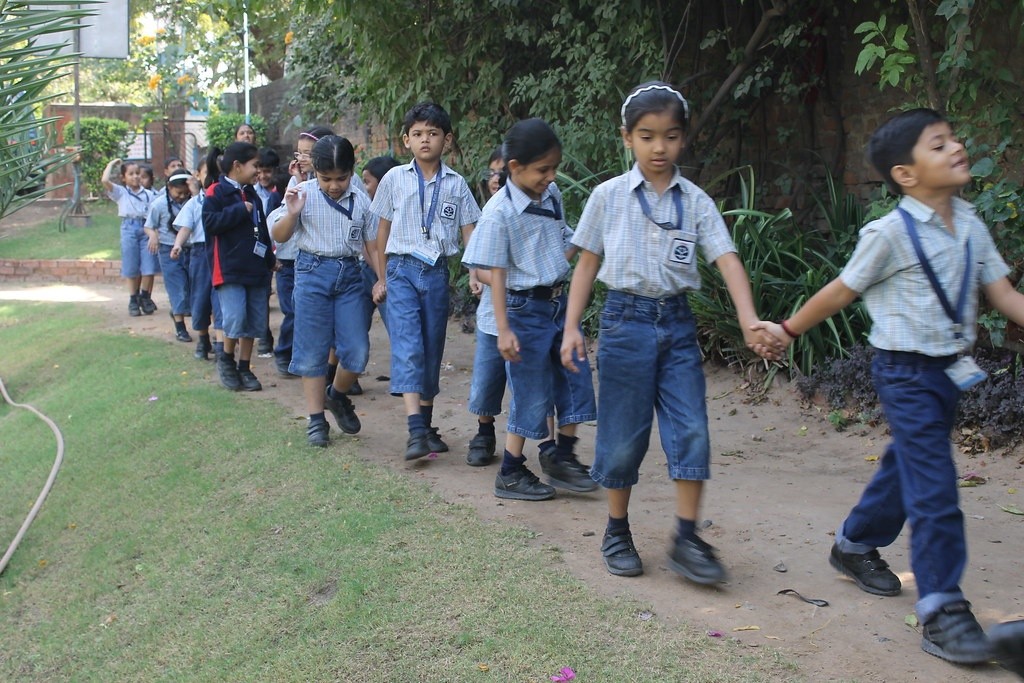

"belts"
[510, 283, 564, 299]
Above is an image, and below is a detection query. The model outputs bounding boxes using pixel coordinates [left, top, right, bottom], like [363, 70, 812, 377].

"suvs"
[0, 88, 40, 169]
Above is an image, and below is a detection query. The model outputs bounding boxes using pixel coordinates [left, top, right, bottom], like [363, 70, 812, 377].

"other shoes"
[988, 620, 1024, 679]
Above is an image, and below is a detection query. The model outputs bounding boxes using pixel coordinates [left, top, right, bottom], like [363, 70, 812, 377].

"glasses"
[484, 169, 506, 181]
[294, 151, 311, 159]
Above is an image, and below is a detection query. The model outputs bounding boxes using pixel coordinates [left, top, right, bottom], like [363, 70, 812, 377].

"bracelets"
[780, 319, 800, 338]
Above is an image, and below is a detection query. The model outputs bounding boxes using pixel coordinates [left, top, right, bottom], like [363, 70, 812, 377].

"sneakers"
[921, 600, 995, 663]
[405, 429, 431, 460]
[176, 325, 192, 342]
[667, 534, 724, 584]
[194, 341, 212, 360]
[274, 351, 302, 377]
[829, 543, 901, 596]
[324, 385, 361, 434]
[425, 426, 448, 452]
[538, 446, 599, 492]
[217, 356, 241, 391]
[600, 524, 644, 576]
[129, 303, 141, 316]
[237, 368, 262, 391]
[306, 418, 331, 447]
[257, 336, 274, 354]
[466, 433, 496, 467]
[140, 299, 157, 313]
[210, 341, 226, 360]
[349, 380, 363, 395]
[493, 465, 556, 501]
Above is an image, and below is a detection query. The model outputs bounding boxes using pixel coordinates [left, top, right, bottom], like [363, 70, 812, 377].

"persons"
[748, 108, 1024, 664]
[271, 134, 390, 447]
[467, 145, 588, 473]
[102, 123, 401, 394]
[370, 102, 484, 461]
[560, 81, 785, 583]
[460, 118, 597, 499]
